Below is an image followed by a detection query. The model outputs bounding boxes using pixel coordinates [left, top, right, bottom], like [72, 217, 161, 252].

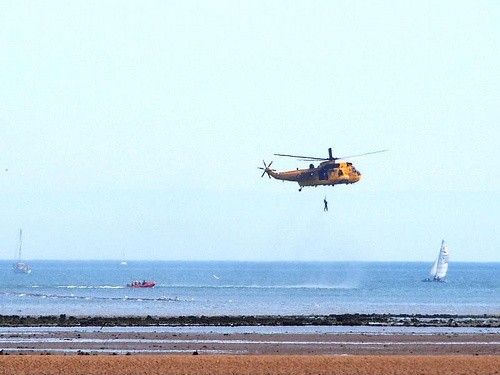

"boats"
[126, 280, 156, 288]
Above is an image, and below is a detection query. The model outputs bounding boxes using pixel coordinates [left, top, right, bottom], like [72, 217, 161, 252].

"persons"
[323, 199, 329, 211]
[132, 280, 147, 287]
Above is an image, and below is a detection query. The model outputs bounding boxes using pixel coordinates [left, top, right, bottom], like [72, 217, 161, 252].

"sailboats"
[419, 240, 448, 284]
[10, 229, 32, 274]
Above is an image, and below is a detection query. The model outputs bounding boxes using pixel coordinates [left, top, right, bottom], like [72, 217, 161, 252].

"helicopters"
[257, 147, 390, 192]
